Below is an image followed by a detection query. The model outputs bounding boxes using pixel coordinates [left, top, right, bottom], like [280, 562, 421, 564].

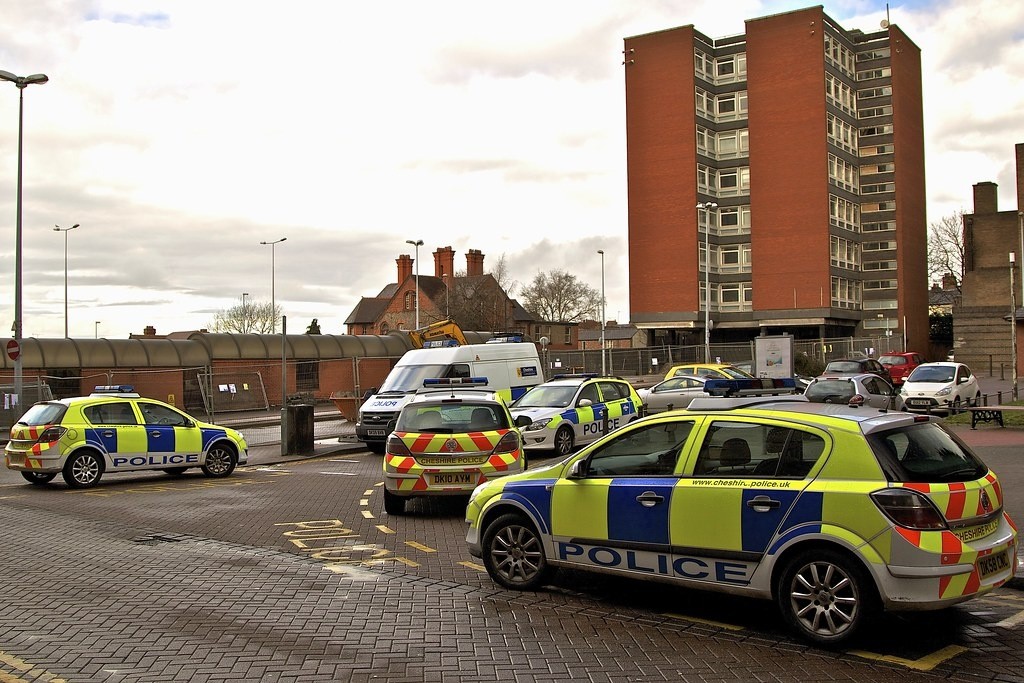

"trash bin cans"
[286, 404, 314, 453]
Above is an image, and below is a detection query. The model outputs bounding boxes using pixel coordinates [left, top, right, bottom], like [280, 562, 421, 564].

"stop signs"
[6, 340, 19, 360]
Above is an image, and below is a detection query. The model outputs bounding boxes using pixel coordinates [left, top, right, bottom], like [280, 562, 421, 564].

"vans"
[355, 342, 545, 455]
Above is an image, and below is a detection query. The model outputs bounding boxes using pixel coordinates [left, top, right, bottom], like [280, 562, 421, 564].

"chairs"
[471, 408, 494, 426]
[753, 429, 803, 475]
[421, 411, 441, 424]
[720, 438, 751, 474]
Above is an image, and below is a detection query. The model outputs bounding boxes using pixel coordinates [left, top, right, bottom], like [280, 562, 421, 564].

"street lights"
[0, 69, 49, 422]
[598, 250, 605, 379]
[696, 202, 717, 364]
[406, 240, 424, 329]
[54, 224, 81, 338]
[260, 238, 286, 334]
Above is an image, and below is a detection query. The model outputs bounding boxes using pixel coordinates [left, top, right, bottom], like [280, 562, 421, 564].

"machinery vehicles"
[408, 318, 468, 349]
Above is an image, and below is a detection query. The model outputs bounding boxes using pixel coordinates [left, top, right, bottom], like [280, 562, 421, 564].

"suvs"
[506, 375, 643, 456]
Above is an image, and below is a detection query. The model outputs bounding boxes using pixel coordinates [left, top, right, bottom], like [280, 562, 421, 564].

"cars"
[4, 393, 248, 490]
[466, 395, 1018, 649]
[636, 376, 725, 413]
[876, 352, 930, 385]
[900, 362, 981, 415]
[382, 391, 528, 513]
[803, 373, 908, 412]
[822, 358, 893, 386]
[663, 364, 813, 395]
[946, 350, 955, 362]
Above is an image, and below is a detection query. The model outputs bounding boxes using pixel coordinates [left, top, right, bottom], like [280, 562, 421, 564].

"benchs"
[967, 406, 1024, 430]
